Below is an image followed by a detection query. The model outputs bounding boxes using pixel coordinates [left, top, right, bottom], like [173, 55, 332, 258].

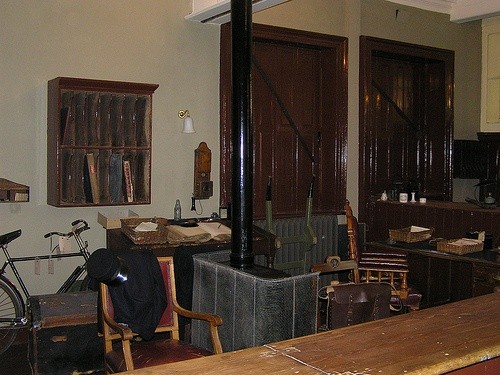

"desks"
[366, 239, 500, 307]
[264, 291, 500, 375]
[107, 216, 276, 327]
[107, 345, 327, 375]
[369, 199, 500, 245]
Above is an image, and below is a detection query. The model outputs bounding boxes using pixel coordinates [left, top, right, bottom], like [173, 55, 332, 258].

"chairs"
[100, 257, 223, 375]
[345, 200, 422, 312]
[319, 282, 403, 331]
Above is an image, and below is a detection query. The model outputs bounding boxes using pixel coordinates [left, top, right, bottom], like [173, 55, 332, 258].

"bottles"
[381, 193, 387, 201]
[174, 200, 181, 220]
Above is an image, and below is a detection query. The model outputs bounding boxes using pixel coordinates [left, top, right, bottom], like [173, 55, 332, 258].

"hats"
[87, 248, 128, 290]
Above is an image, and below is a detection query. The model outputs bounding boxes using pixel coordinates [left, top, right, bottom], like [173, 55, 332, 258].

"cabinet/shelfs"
[47, 77, 159, 207]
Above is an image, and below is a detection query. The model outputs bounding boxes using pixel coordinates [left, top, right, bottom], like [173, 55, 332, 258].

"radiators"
[254, 214, 340, 288]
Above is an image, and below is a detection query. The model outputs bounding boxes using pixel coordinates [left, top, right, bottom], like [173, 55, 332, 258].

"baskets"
[120, 217, 167, 245]
[389, 226, 434, 244]
[437, 238, 484, 255]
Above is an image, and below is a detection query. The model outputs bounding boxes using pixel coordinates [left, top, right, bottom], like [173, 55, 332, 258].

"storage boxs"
[29, 290, 186, 375]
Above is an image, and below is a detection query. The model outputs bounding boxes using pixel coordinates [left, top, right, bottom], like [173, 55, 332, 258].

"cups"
[399, 193, 408, 203]
[387, 237, 396, 244]
[484, 191, 496, 204]
[420, 198, 426, 203]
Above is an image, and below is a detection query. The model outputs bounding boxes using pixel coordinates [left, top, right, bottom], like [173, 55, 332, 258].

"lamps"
[178, 110, 196, 135]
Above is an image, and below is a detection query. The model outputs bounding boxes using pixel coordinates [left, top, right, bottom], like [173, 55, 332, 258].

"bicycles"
[0, 219, 120, 354]
